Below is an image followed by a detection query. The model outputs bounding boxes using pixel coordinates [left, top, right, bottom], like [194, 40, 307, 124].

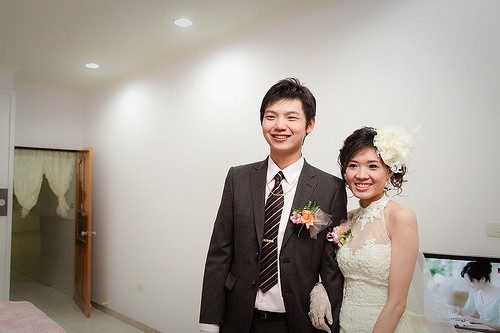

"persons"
[309, 128, 456, 332]
[200, 78, 348, 333]
[441, 261, 500, 330]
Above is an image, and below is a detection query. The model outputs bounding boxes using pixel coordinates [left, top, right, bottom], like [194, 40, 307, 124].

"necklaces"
[267, 179, 298, 196]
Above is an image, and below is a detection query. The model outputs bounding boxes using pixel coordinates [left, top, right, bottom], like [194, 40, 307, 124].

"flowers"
[325, 219, 355, 249]
[289, 200, 334, 241]
[373, 125, 415, 174]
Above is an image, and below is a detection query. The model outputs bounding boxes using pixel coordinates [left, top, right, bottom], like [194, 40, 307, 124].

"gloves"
[308, 283, 333, 333]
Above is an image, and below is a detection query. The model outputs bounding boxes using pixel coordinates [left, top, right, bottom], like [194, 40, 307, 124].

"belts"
[253, 311, 286, 320]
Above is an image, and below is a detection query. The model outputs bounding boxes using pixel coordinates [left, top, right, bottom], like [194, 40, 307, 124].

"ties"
[258, 171, 285, 294]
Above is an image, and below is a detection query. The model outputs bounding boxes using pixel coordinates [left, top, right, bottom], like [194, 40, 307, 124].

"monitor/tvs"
[423, 252, 499, 332]
